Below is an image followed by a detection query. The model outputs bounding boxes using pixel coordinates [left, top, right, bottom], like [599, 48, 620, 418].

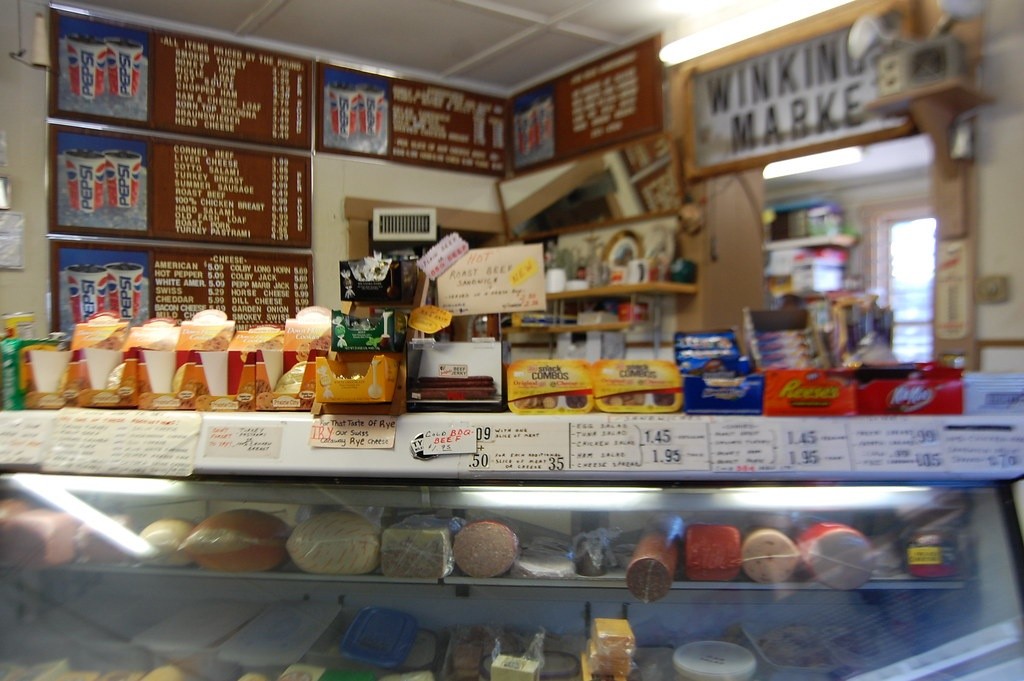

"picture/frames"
[506, 32, 663, 176]
[48, 6, 314, 152]
[313, 60, 513, 177]
[47, 235, 314, 339]
[677, 1, 933, 181]
[43, 122, 314, 248]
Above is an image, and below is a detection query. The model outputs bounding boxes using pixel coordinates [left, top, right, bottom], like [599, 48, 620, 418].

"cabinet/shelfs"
[498, 213, 704, 362]
[0, 467, 1024, 681]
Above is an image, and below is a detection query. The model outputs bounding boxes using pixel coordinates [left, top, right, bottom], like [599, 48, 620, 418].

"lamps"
[658, 1, 850, 67]
